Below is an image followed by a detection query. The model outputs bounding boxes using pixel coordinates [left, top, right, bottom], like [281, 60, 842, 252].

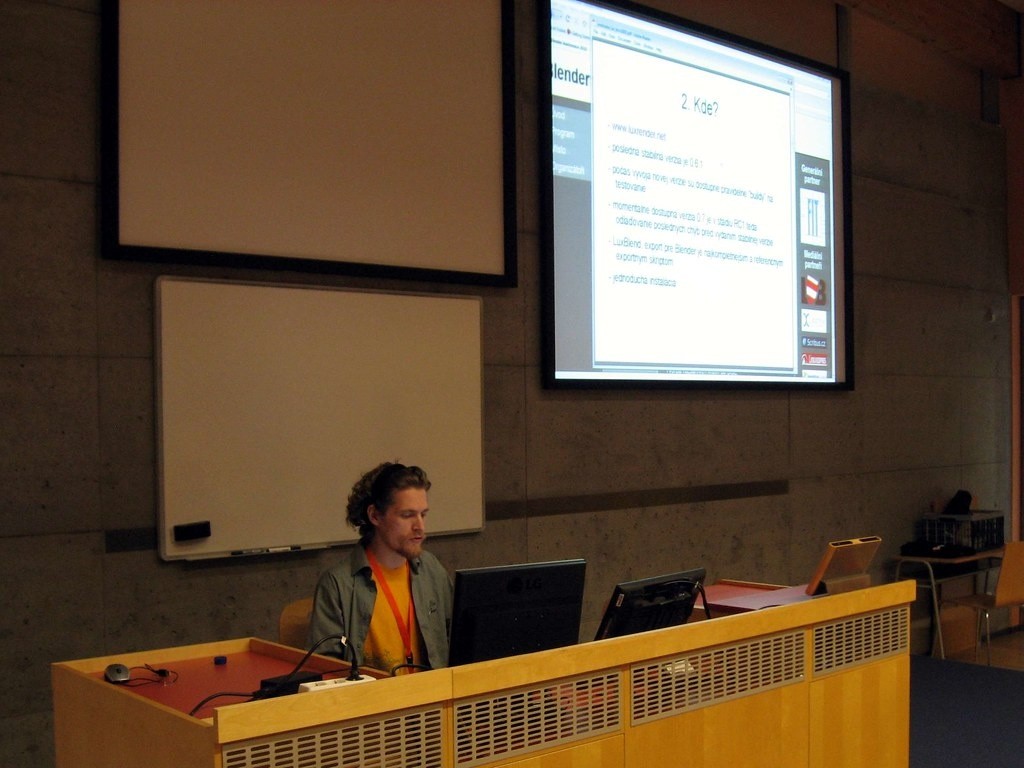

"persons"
[301, 461, 450, 686]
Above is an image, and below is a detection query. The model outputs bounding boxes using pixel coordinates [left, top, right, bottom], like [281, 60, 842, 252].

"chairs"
[276, 597, 320, 653]
[932, 541, 1023, 666]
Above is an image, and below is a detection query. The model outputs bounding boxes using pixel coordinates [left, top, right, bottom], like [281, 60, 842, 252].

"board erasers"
[172, 519, 213, 545]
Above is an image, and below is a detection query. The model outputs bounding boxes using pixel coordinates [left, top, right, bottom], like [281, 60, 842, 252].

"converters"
[257, 671, 322, 696]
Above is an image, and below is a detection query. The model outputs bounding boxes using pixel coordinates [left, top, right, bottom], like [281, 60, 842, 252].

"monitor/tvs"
[447, 558, 586, 668]
[593, 567, 706, 641]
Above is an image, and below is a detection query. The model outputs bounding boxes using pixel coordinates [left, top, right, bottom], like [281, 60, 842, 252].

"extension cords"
[297, 674, 376, 693]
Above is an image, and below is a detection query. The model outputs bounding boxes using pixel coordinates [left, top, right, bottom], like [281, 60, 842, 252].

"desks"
[47, 574, 918, 767]
[886, 541, 1006, 659]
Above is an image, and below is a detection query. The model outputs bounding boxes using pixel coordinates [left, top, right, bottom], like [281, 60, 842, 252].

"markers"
[267, 546, 302, 553]
[231, 549, 265, 556]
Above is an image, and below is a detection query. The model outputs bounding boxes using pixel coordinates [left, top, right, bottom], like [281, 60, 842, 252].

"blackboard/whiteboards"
[155, 274, 486, 570]
